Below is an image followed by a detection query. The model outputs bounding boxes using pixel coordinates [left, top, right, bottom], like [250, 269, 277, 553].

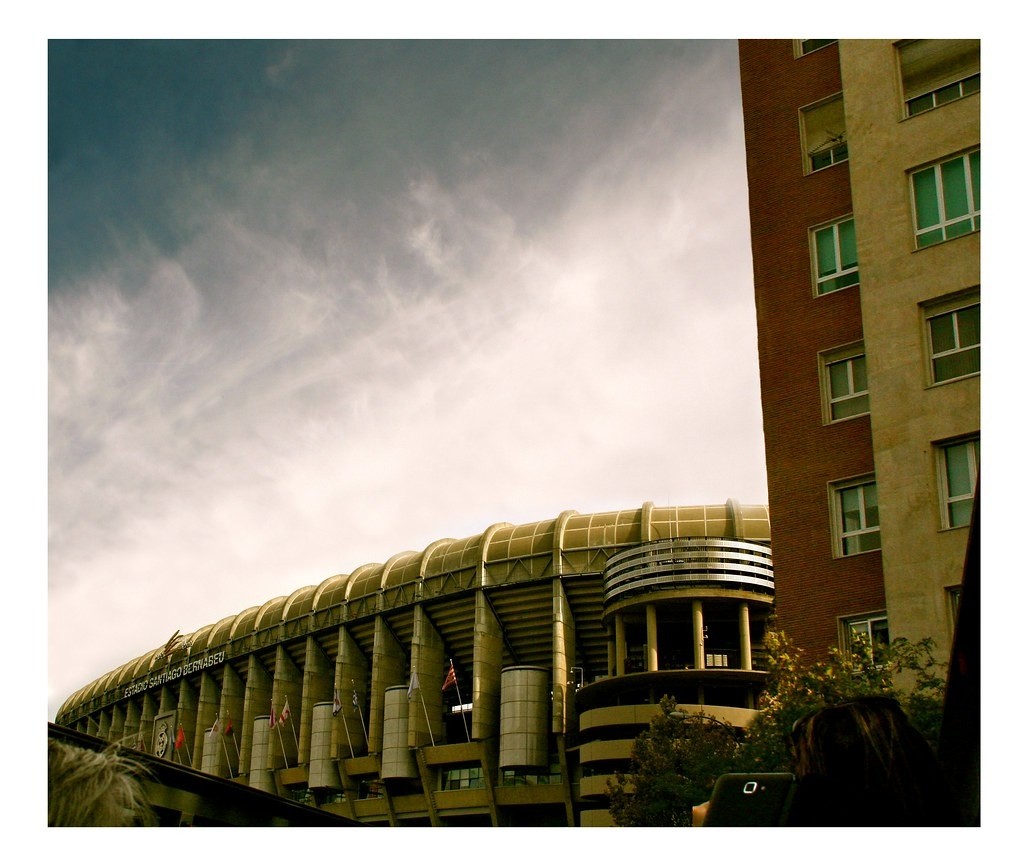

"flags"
[279, 701, 289, 728]
[353, 687, 358, 711]
[269, 707, 277, 729]
[225, 717, 233, 736]
[408, 672, 419, 700]
[170, 727, 184, 751]
[210, 720, 220, 738]
[442, 664, 456, 691]
[332, 689, 342, 716]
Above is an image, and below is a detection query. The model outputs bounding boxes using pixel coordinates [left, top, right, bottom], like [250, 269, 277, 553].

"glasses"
[791, 706, 857, 767]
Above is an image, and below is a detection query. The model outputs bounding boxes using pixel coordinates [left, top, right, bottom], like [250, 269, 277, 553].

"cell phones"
[700, 771, 797, 828]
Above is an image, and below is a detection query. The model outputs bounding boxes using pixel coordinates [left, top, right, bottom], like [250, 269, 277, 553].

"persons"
[790, 695, 936, 826]
[48, 739, 158, 827]
